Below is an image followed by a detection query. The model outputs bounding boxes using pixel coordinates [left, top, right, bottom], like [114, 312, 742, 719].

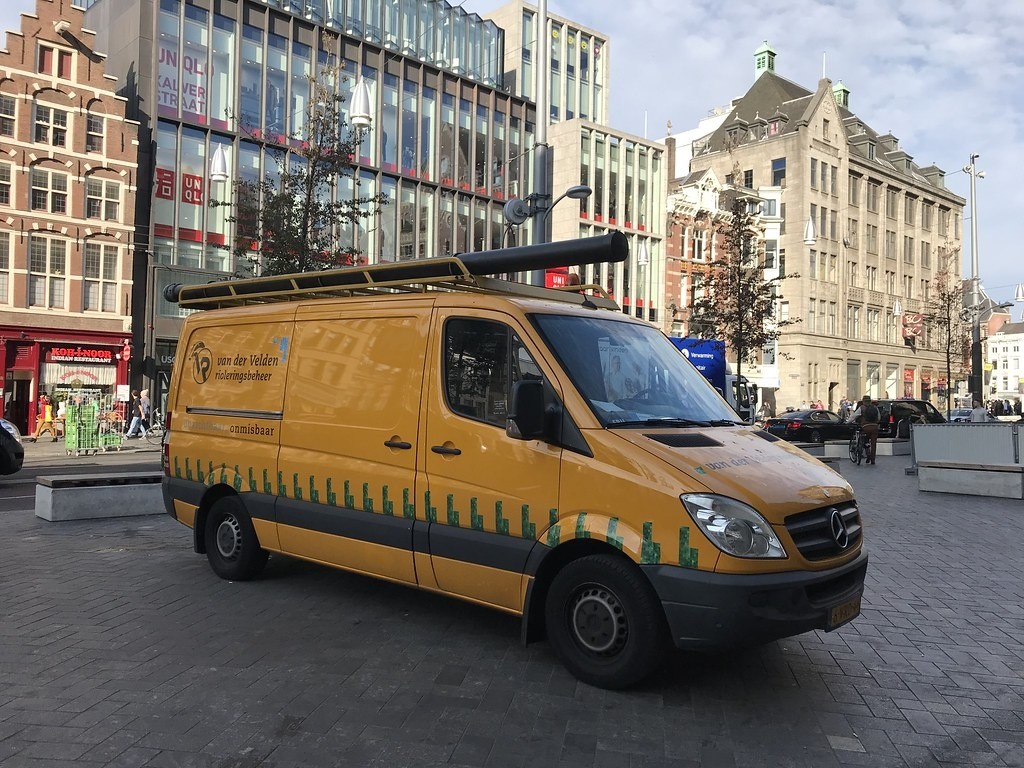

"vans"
[161, 233, 872, 692]
[853, 400, 945, 438]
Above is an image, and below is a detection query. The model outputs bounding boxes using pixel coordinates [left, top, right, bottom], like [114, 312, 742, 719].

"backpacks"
[864, 404, 878, 422]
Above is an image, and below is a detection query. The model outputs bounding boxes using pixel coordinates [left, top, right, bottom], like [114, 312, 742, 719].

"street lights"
[971, 301, 1015, 408]
[345, 74, 374, 268]
[529, 183, 592, 288]
[964, 150, 987, 408]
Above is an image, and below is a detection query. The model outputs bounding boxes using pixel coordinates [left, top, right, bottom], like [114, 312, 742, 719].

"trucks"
[596, 336, 758, 426]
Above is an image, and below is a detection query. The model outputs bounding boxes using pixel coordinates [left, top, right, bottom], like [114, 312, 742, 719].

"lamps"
[54, 19, 72, 36]
[804, 215, 817, 246]
[892, 298, 902, 316]
[637, 237, 650, 266]
[1015, 283, 1024, 322]
[350, 75, 373, 130]
[209, 141, 229, 183]
[20, 332, 30, 340]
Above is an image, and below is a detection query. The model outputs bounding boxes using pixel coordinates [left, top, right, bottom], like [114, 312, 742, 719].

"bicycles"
[848, 426, 863, 465]
[145, 407, 166, 446]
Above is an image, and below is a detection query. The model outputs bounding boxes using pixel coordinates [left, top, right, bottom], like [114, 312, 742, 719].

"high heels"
[51, 438, 57, 442]
[29, 438, 36, 443]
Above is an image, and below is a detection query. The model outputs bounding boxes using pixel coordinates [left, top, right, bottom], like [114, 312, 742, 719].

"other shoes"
[866, 455, 872, 463]
[139, 436, 146, 440]
[871, 458, 875, 464]
[123, 435, 128, 440]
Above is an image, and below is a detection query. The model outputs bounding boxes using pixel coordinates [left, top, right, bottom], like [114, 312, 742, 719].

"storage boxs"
[65, 405, 123, 448]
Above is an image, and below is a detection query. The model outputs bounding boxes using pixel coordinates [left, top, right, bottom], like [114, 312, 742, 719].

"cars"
[946, 410, 1001, 421]
[0, 417, 25, 476]
[764, 409, 847, 444]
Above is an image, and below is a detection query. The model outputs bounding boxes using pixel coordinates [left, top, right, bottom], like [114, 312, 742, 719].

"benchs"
[812, 456, 841, 475]
[33, 471, 168, 521]
[824, 437, 911, 457]
[912, 460, 1024, 500]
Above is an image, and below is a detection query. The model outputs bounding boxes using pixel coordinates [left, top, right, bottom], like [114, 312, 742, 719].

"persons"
[132, 389, 155, 438]
[29, 392, 59, 443]
[985, 397, 1022, 416]
[844, 394, 880, 465]
[610, 354, 628, 402]
[839, 396, 858, 421]
[124, 389, 148, 440]
[810, 401, 815, 409]
[816, 400, 824, 410]
[801, 401, 808, 410]
[758, 401, 772, 422]
[960, 399, 989, 424]
